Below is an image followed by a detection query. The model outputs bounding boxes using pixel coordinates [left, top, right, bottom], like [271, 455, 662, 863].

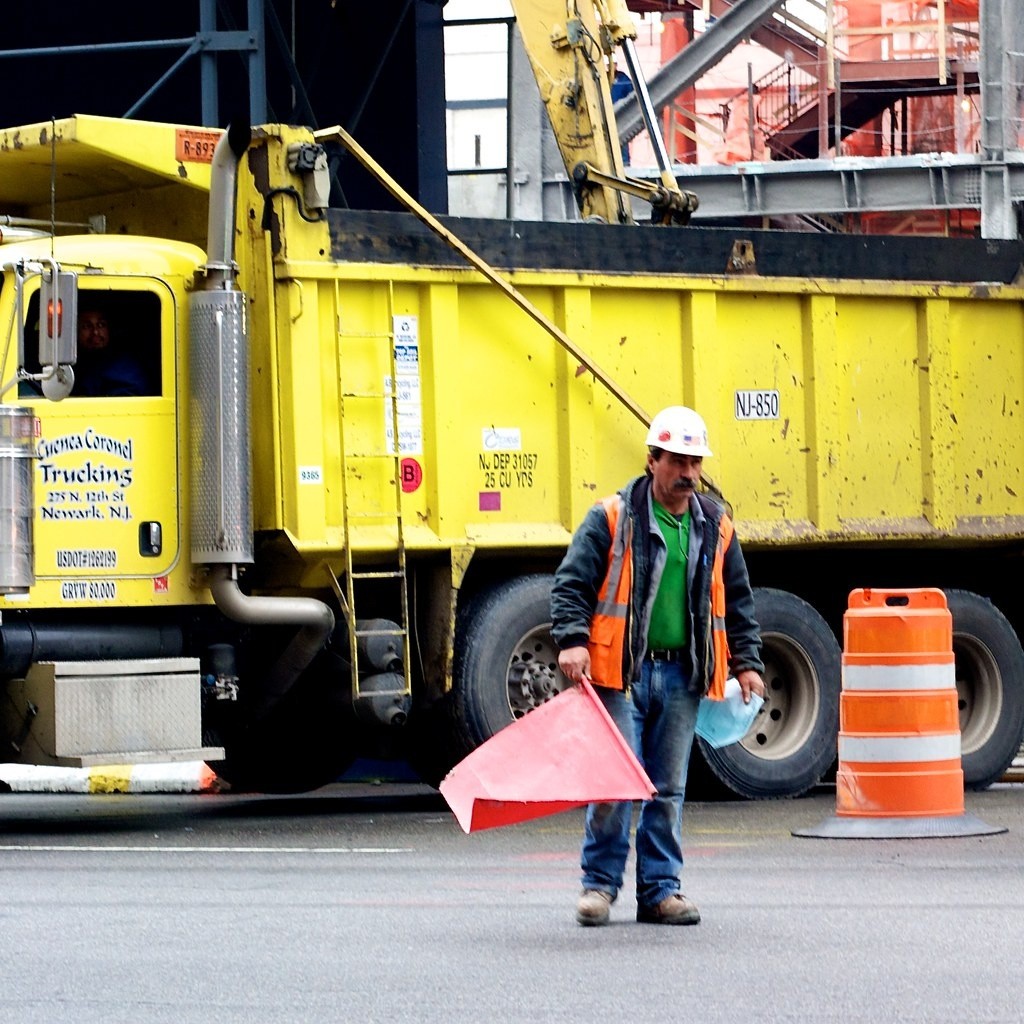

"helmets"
[645, 406, 712, 457]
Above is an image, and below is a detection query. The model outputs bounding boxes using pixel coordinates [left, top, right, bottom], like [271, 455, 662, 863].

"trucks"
[0, 111, 1024, 801]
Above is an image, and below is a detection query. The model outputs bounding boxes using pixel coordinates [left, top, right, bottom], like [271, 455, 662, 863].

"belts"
[644, 647, 686, 662]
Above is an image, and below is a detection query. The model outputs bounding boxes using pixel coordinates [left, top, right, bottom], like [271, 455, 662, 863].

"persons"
[77, 309, 147, 395]
[605, 52, 634, 167]
[550, 406, 765, 924]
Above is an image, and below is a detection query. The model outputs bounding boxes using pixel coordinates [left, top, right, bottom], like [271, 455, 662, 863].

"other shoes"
[577, 884, 614, 926]
[637, 893, 700, 926]
